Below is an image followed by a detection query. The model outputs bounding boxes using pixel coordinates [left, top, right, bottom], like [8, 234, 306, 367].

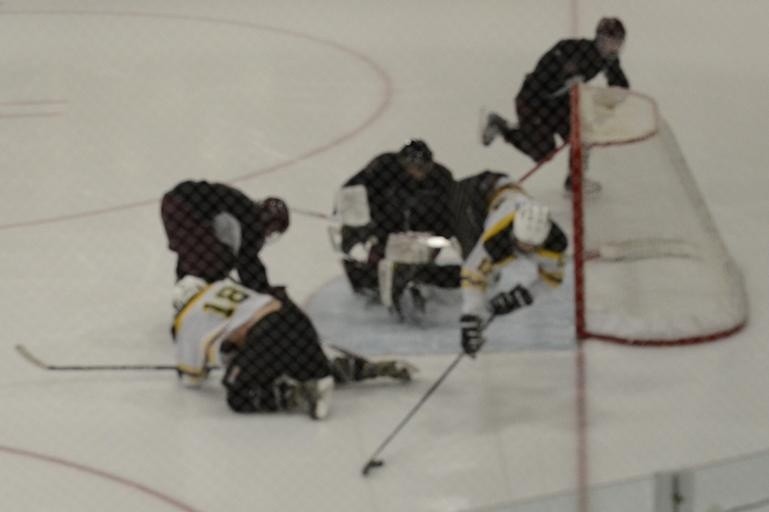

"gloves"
[487, 284, 533, 314]
[459, 314, 485, 359]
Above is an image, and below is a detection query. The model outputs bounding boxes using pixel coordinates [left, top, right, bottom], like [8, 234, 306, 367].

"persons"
[169, 275, 415, 420]
[482, 17, 632, 197]
[332, 139, 459, 310]
[161, 181, 289, 291]
[389, 171, 569, 357]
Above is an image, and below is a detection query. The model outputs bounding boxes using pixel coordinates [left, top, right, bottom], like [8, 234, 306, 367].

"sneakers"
[391, 261, 427, 315]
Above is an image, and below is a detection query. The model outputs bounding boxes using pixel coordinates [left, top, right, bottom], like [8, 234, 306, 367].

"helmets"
[396, 139, 433, 174]
[513, 203, 552, 257]
[595, 18, 626, 63]
[171, 274, 207, 310]
[261, 197, 289, 237]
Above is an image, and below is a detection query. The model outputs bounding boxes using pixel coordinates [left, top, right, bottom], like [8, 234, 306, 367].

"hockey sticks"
[16, 345, 179, 370]
[363, 311, 496, 472]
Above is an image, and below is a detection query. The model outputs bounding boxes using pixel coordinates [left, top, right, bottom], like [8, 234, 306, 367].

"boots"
[484, 113, 506, 146]
[376, 360, 411, 380]
[565, 173, 601, 195]
[296, 378, 318, 418]
